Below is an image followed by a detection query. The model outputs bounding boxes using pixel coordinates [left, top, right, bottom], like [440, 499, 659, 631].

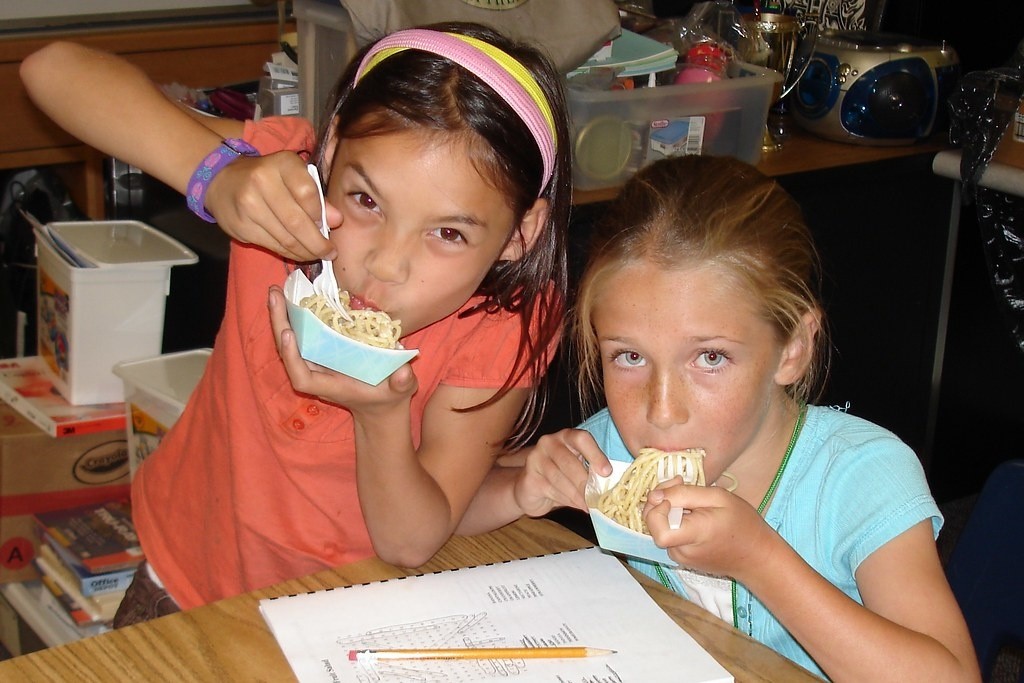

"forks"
[656, 454, 695, 529]
[307, 164, 354, 323]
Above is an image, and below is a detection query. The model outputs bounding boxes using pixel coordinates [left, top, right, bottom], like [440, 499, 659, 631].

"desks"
[571, 132, 952, 205]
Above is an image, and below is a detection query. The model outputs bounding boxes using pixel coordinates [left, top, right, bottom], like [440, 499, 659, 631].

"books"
[0, 498, 146, 646]
[0, 356, 126, 438]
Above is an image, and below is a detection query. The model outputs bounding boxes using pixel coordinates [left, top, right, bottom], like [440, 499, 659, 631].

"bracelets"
[186, 137, 261, 223]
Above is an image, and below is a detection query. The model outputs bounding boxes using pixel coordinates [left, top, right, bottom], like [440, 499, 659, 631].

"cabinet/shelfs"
[0, 9, 302, 360]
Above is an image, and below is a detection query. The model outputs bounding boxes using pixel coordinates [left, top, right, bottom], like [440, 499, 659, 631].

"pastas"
[598, 448, 737, 537]
[299, 291, 402, 349]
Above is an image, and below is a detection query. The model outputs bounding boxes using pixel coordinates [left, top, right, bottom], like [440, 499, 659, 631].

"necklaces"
[653, 406, 802, 630]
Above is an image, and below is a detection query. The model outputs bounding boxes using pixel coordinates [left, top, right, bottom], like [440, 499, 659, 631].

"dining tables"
[0, 515, 825, 683]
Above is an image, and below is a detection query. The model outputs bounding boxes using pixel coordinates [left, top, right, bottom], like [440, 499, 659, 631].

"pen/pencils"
[348, 645, 616, 661]
[638, 72, 656, 163]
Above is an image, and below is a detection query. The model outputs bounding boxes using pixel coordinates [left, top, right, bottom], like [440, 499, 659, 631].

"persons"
[21, 21, 571, 634]
[453, 154, 984, 683]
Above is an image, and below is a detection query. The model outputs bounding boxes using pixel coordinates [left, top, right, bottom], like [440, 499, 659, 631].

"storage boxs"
[294, 0, 364, 137]
[0, 579, 87, 655]
[32, 222, 199, 407]
[108, 348, 213, 484]
[0, 400, 132, 512]
[563, 60, 787, 194]
